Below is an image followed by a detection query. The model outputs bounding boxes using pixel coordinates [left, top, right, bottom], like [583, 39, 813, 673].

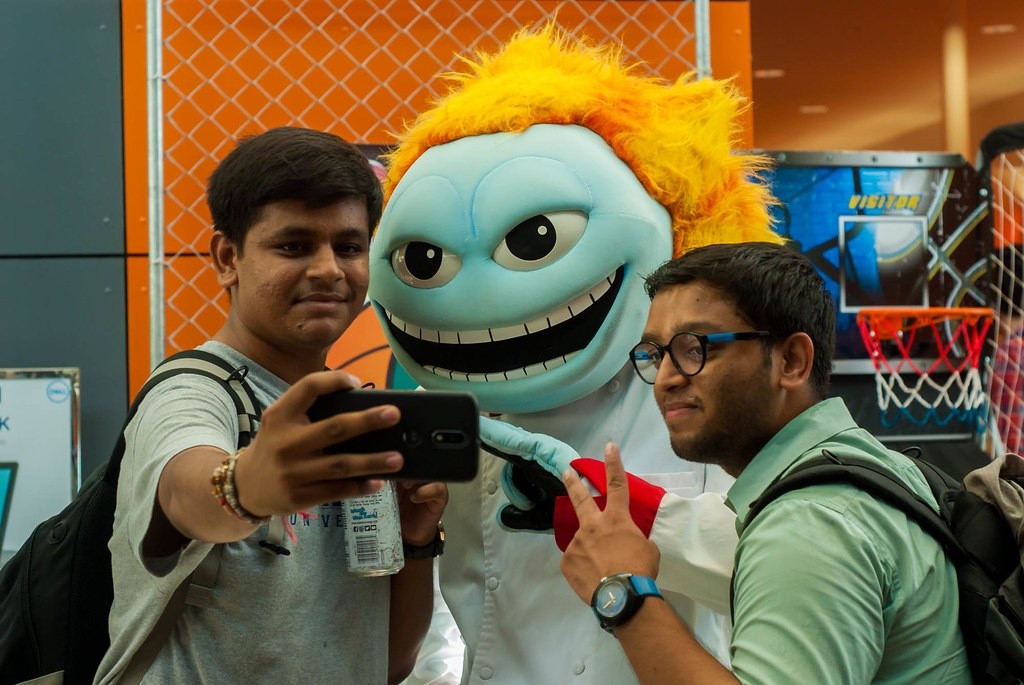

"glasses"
[627, 331, 783, 385]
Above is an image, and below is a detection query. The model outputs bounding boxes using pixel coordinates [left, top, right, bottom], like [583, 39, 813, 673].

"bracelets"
[210, 448, 276, 525]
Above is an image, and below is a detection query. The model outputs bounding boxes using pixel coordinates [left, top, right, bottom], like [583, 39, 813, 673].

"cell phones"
[304, 389, 480, 485]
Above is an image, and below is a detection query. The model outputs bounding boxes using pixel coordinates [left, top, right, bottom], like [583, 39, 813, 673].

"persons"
[91, 126, 449, 685]
[559, 241, 972, 685]
[992, 243, 1024, 459]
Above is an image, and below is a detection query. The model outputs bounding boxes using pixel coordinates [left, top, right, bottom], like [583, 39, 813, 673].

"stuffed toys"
[365, 5, 796, 685]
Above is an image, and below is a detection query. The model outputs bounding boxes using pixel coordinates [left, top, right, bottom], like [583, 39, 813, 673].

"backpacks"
[0, 349, 265, 685]
[729, 449, 1024, 685]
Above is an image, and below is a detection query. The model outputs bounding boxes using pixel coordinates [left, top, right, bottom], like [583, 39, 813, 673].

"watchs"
[402, 520, 446, 561]
[590, 573, 663, 638]
